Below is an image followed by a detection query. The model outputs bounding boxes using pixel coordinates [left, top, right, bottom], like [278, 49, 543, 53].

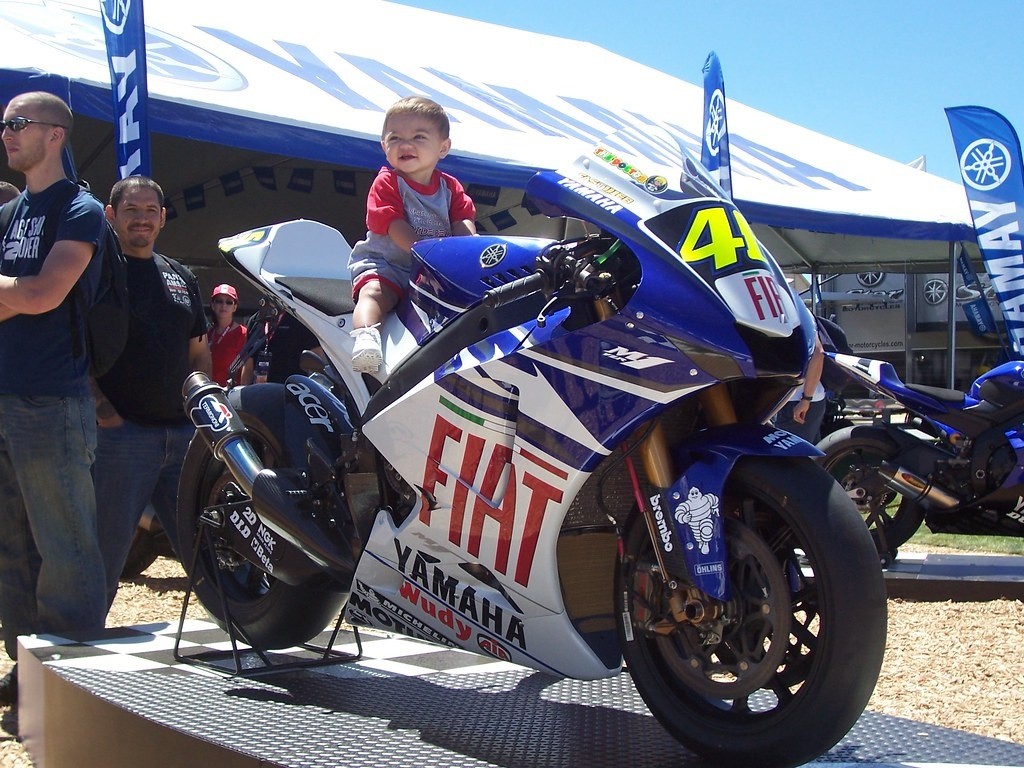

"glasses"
[0, 116, 69, 133]
[213, 298, 235, 305]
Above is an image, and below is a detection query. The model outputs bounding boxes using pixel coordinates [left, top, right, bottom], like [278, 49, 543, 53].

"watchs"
[801, 392, 813, 402]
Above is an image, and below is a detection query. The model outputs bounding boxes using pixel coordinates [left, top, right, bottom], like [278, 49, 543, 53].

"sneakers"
[350, 322, 382, 374]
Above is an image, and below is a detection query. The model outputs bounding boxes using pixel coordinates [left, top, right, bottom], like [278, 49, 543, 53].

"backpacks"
[0, 180, 131, 378]
[815, 315, 853, 393]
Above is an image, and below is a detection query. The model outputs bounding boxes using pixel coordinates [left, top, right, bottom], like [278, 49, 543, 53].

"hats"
[210, 283, 238, 301]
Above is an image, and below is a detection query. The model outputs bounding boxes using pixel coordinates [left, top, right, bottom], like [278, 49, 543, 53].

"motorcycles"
[175, 124, 889, 768]
[811, 349, 1023, 559]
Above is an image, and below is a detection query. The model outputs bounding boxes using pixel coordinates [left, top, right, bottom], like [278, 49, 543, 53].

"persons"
[347, 96, 481, 374]
[772, 287, 828, 445]
[0, 91, 251, 706]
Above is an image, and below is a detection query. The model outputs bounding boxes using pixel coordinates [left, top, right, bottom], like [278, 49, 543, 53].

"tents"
[0, 0, 988, 391]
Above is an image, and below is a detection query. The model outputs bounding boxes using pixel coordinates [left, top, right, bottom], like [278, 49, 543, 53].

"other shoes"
[0, 662, 17, 707]
